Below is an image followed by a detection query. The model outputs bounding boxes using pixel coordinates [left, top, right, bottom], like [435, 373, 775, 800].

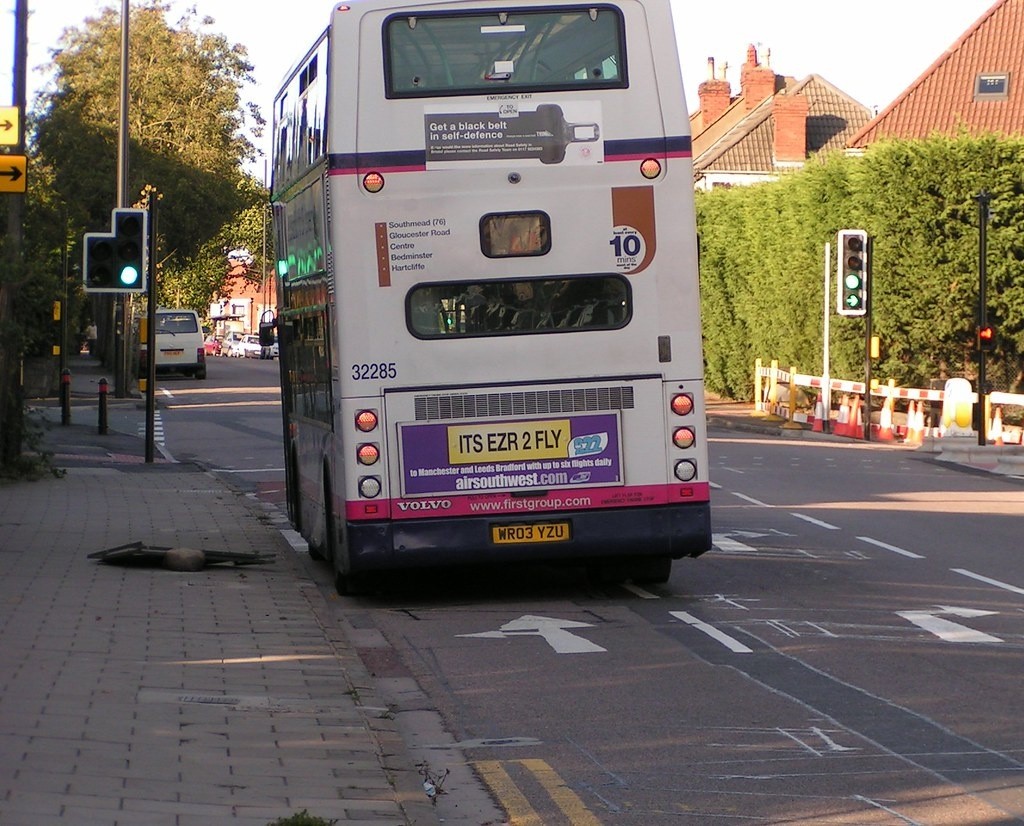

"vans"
[139, 309, 207, 378]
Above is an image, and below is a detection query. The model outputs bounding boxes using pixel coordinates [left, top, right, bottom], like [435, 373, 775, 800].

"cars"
[205, 334, 226, 357]
[238, 334, 263, 359]
[220, 330, 246, 359]
[261, 335, 279, 360]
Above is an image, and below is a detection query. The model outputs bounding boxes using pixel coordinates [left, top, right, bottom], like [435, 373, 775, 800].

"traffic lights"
[115, 208, 148, 292]
[836, 229, 867, 317]
[977, 323, 998, 354]
[83, 232, 117, 293]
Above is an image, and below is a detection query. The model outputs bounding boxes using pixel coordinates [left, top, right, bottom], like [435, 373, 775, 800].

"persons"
[85, 320, 97, 356]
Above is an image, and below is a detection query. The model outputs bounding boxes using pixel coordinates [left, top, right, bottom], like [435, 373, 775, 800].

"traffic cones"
[845, 395, 859, 438]
[857, 403, 865, 441]
[993, 406, 1007, 447]
[910, 401, 925, 448]
[874, 397, 895, 440]
[833, 394, 850, 436]
[812, 392, 822, 432]
[902, 400, 916, 443]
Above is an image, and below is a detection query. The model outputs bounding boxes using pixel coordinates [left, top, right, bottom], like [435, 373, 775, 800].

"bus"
[261, 0, 713, 598]
